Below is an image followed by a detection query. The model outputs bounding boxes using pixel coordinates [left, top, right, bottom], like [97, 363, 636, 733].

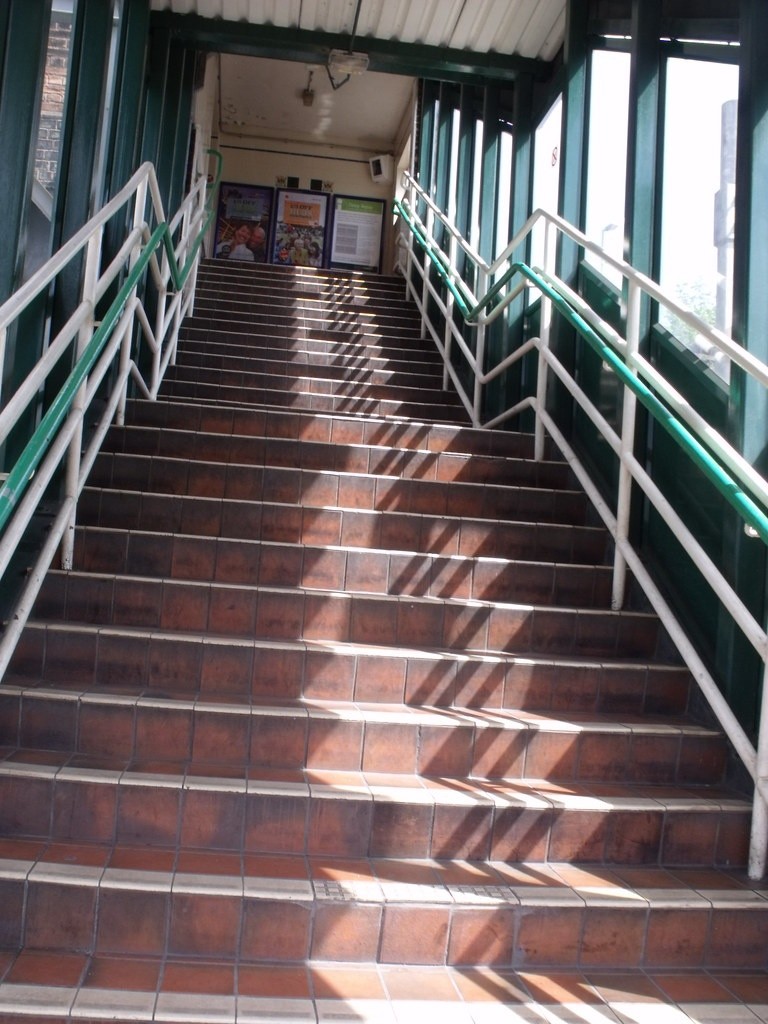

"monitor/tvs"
[369, 154, 394, 182]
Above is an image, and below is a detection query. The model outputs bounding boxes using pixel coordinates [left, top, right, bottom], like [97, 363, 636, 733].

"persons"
[214, 224, 265, 262]
[274, 232, 323, 267]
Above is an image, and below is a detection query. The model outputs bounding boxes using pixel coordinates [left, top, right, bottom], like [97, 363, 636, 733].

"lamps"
[303, 90, 314, 107]
